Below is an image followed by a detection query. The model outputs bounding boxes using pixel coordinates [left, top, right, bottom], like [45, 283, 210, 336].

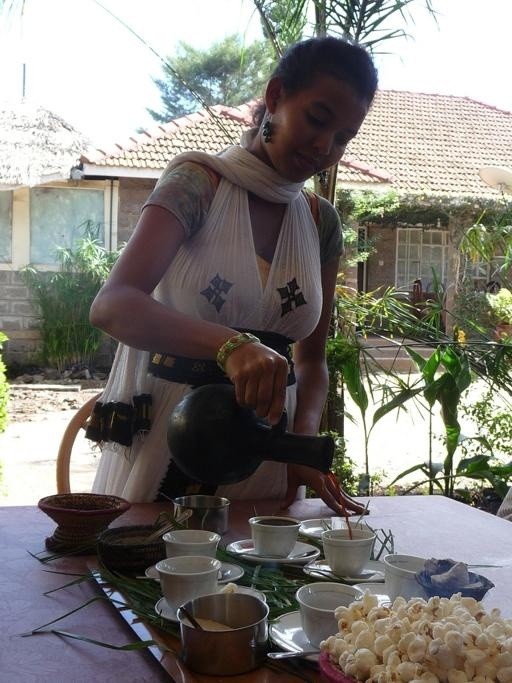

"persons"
[89, 37, 378, 518]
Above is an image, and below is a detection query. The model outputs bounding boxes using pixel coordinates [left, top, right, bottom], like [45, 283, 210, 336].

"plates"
[227, 538, 319, 564]
[269, 611, 341, 669]
[156, 587, 267, 626]
[304, 560, 392, 582]
[146, 558, 246, 585]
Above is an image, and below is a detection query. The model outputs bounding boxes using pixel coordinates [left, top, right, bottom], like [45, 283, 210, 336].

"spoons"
[267, 648, 324, 660]
[178, 603, 207, 635]
[159, 493, 182, 505]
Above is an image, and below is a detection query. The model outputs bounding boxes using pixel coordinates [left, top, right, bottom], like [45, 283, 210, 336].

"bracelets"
[216, 329, 260, 373]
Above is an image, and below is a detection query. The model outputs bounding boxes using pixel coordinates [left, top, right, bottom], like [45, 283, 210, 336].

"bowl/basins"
[420, 568, 494, 606]
[173, 496, 229, 535]
[178, 592, 269, 676]
[99, 522, 171, 578]
[319, 644, 356, 683]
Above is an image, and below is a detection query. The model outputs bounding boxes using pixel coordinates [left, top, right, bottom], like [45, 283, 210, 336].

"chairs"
[55, 387, 309, 501]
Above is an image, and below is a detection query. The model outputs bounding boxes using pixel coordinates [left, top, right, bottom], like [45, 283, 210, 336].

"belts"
[151, 326, 296, 387]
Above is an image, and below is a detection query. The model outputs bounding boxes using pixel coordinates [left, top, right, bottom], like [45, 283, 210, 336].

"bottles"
[167, 382, 335, 485]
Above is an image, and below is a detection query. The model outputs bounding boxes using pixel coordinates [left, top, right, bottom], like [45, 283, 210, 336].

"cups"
[322, 529, 374, 574]
[297, 582, 364, 647]
[161, 529, 219, 560]
[381, 555, 432, 600]
[156, 555, 220, 605]
[247, 517, 300, 558]
